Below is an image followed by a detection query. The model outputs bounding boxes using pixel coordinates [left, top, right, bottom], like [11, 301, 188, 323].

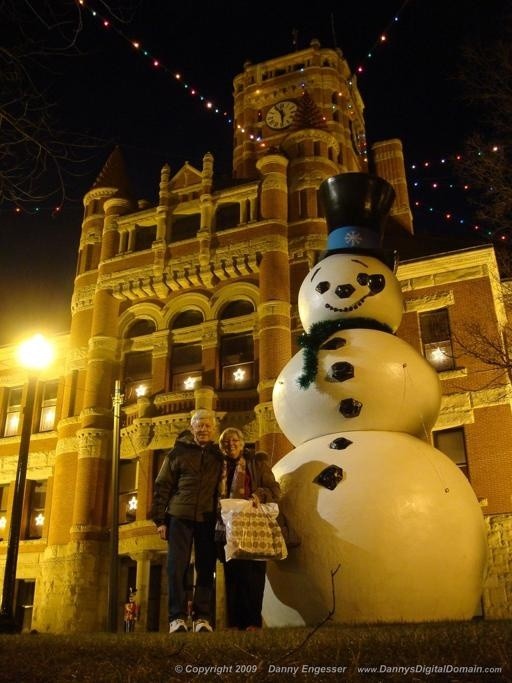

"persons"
[122, 596, 141, 633]
[150, 408, 226, 635]
[214, 426, 308, 634]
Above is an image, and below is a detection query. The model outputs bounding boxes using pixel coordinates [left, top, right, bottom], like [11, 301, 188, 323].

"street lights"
[0, 330, 59, 635]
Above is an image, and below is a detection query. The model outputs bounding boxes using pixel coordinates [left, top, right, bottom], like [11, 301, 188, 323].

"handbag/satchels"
[219, 497, 289, 563]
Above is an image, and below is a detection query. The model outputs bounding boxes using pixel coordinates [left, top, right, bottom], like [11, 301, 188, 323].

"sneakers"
[169, 618, 188, 634]
[192, 618, 213, 633]
[227, 624, 258, 633]
